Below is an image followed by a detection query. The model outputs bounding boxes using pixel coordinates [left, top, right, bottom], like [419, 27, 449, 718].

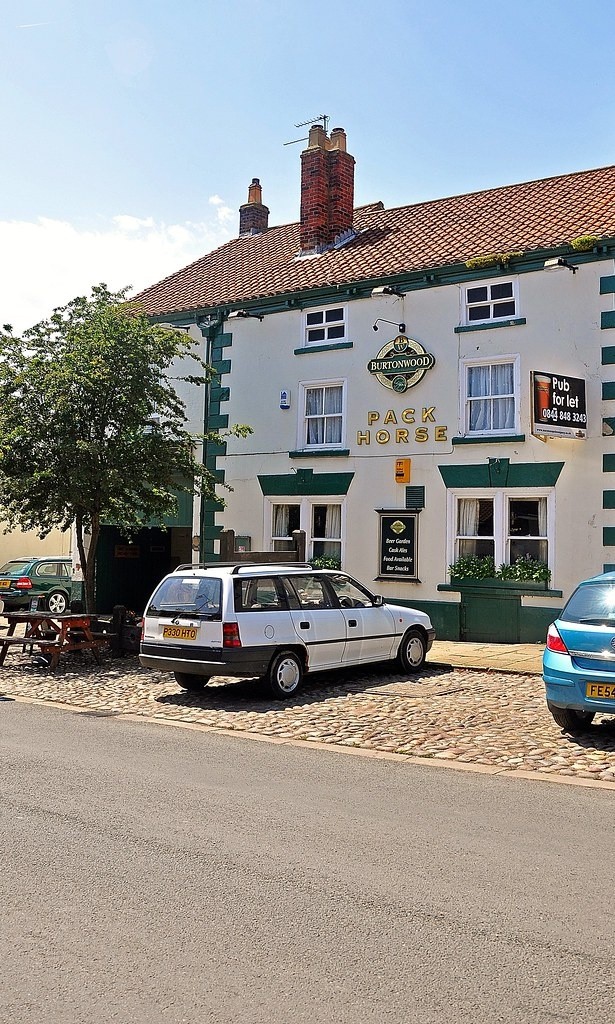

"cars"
[0, 555, 74, 613]
[541, 571, 615, 733]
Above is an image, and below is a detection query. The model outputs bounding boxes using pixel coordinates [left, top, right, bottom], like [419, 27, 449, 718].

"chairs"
[41, 566, 55, 575]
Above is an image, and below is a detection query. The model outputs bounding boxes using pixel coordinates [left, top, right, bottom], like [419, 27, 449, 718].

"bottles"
[30, 597, 38, 613]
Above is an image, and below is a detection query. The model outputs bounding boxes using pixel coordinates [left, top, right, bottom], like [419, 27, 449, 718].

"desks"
[0, 611, 105, 672]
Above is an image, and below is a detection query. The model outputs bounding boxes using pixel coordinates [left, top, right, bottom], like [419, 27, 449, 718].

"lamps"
[228, 309, 264, 322]
[152, 323, 190, 333]
[543, 259, 578, 276]
[372, 318, 406, 334]
[371, 284, 407, 300]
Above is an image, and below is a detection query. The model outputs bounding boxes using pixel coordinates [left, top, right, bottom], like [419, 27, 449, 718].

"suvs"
[139, 561, 436, 698]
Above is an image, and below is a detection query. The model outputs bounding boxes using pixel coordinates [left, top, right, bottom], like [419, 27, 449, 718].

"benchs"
[38, 630, 116, 647]
[0, 636, 60, 654]
